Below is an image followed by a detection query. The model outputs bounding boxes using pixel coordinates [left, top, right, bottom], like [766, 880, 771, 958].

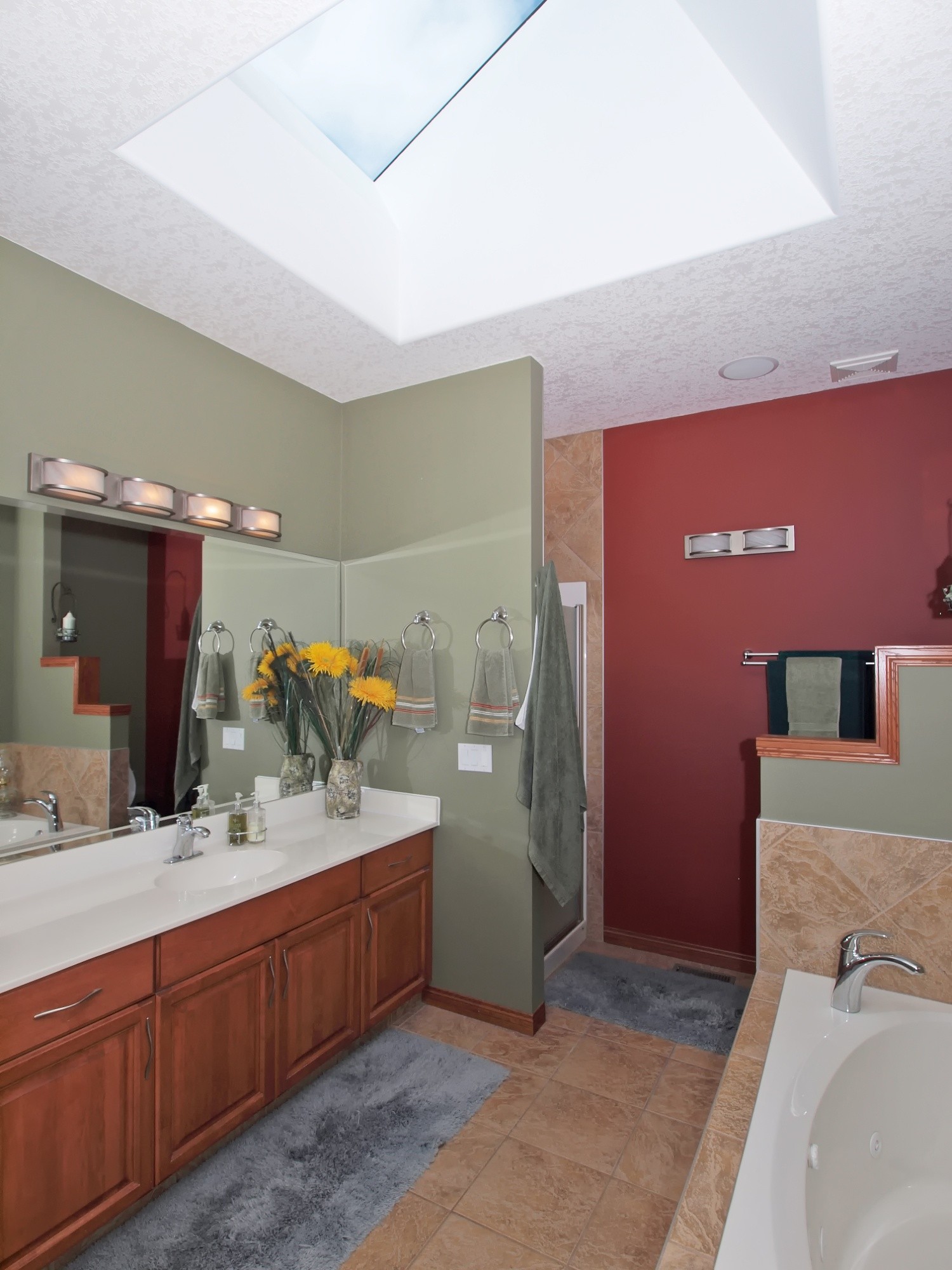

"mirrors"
[0, 496, 340, 868]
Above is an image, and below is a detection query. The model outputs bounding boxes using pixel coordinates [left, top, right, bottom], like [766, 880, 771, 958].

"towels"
[515, 560, 586, 905]
[776, 649, 873, 738]
[191, 651, 226, 720]
[765, 657, 866, 740]
[248, 648, 273, 722]
[391, 647, 437, 734]
[173, 594, 204, 812]
[465, 648, 520, 737]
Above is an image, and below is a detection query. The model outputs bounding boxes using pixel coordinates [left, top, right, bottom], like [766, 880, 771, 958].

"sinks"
[154, 847, 287, 893]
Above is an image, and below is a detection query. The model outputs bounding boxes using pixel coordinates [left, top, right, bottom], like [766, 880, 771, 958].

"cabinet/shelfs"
[360, 828, 433, 1027]
[0, 937, 155, 1270]
[155, 855, 360, 1187]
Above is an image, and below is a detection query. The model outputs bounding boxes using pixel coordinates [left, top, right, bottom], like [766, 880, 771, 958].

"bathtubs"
[711, 967, 951, 1269]
[0, 820, 100, 853]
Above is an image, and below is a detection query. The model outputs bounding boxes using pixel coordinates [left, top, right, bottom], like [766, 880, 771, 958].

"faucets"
[830, 928, 926, 1013]
[127, 804, 161, 833]
[21, 789, 63, 832]
[163, 813, 211, 864]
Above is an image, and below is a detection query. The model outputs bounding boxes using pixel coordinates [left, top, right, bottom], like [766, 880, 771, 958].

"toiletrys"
[226, 792, 247, 846]
[191, 787, 209, 818]
[200, 784, 215, 815]
[245, 791, 267, 844]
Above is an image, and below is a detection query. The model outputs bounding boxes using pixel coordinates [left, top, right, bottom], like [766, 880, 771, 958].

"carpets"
[542, 951, 753, 1059]
[59, 1026, 512, 1270]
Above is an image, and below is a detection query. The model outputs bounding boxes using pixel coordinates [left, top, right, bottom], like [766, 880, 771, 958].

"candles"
[61, 608, 76, 641]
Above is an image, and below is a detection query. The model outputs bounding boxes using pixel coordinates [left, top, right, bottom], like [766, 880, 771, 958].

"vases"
[277, 752, 316, 799]
[325, 756, 364, 820]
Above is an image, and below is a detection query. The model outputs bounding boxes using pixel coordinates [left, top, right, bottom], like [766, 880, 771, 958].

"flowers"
[242, 634, 317, 756]
[275, 629, 400, 760]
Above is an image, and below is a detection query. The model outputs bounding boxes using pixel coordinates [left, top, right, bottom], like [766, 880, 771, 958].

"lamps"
[28, 451, 282, 543]
[683, 524, 796, 560]
